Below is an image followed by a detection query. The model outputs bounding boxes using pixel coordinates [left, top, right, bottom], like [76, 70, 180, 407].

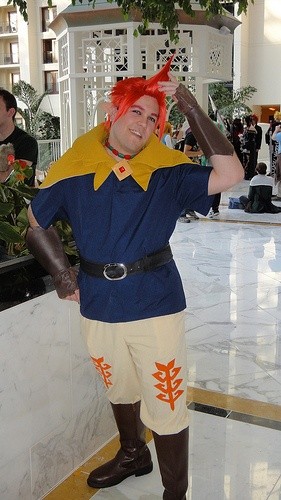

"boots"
[87, 400, 153, 488]
[151, 426, 189, 500]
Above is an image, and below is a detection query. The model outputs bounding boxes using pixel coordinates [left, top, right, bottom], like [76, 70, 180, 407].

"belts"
[80, 244, 173, 280]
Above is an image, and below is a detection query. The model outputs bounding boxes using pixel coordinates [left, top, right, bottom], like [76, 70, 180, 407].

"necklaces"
[105, 139, 143, 161]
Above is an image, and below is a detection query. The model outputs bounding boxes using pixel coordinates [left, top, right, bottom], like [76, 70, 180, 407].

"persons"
[24, 51, 245, 500]
[159, 110, 281, 223]
[0, 90, 45, 299]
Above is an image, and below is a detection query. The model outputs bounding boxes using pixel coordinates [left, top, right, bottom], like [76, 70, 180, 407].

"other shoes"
[177, 217, 190, 223]
[271, 195, 281, 201]
[208, 210, 220, 219]
[187, 211, 199, 220]
